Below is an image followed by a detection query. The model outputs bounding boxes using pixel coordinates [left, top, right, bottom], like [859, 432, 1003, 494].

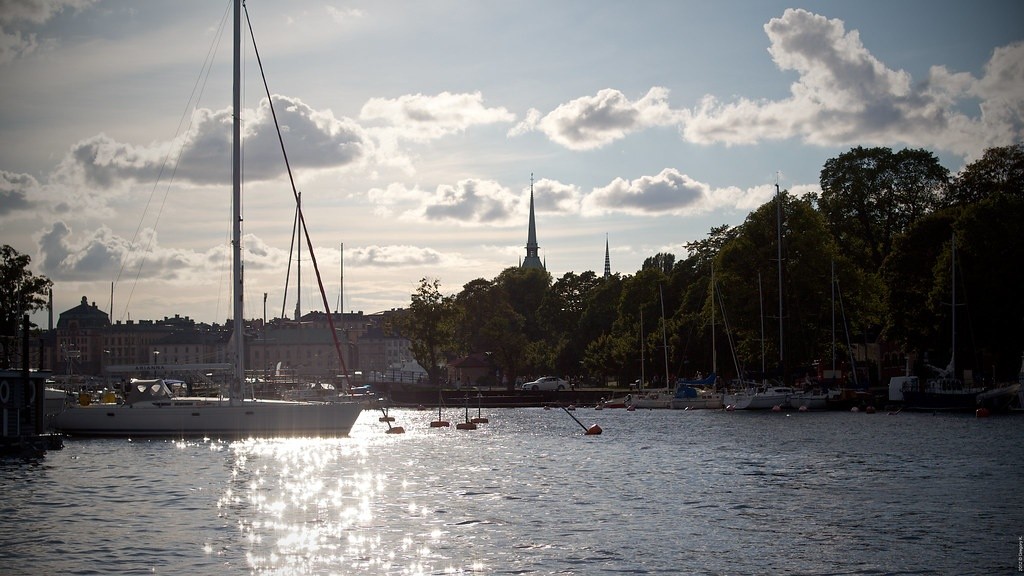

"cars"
[521, 375, 572, 392]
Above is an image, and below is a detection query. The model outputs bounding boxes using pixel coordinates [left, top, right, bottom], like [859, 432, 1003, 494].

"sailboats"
[46, 0, 371, 437]
[595, 184, 1024, 415]
